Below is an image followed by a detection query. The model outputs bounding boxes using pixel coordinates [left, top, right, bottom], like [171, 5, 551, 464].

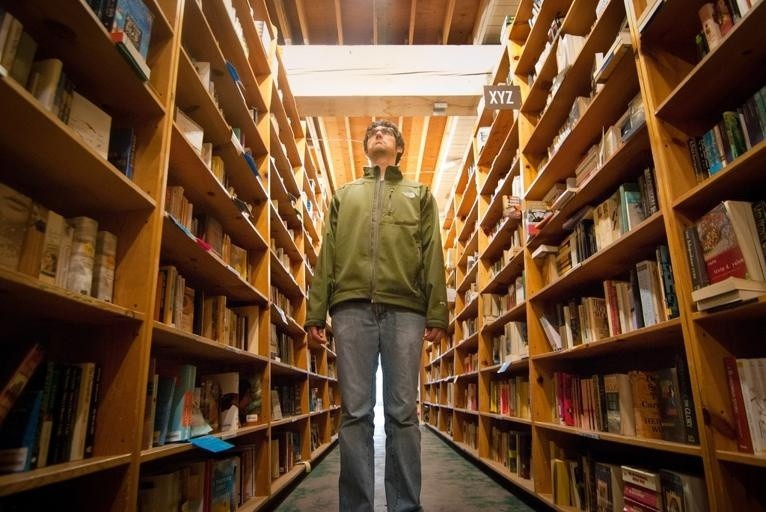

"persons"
[303, 119, 450, 512]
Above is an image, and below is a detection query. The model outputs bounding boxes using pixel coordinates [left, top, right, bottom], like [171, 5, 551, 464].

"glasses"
[366, 126, 398, 138]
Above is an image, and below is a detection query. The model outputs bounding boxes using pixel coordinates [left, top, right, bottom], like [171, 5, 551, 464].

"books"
[144, 441, 259, 512]
[143, 357, 266, 451]
[462, 317, 478, 340]
[0, 182, 119, 305]
[481, 270, 528, 326]
[269, 323, 296, 367]
[270, 284, 296, 320]
[522, 0, 660, 286]
[0, 336, 105, 475]
[464, 281, 477, 306]
[721, 356, 766, 457]
[683, 198, 766, 313]
[89, 0, 157, 81]
[68, 90, 140, 181]
[271, 198, 297, 242]
[154, 263, 250, 352]
[552, 364, 701, 446]
[302, 174, 329, 245]
[0, 9, 73, 126]
[463, 383, 479, 412]
[466, 249, 478, 274]
[271, 428, 302, 480]
[539, 244, 679, 353]
[491, 320, 529, 365]
[461, 419, 479, 450]
[467, 125, 494, 181]
[490, 221, 524, 281]
[489, 424, 531, 481]
[270, 376, 302, 422]
[489, 375, 531, 421]
[173, 0, 272, 218]
[547, 438, 706, 512]
[165, 184, 248, 280]
[463, 352, 479, 374]
[693, 0, 760, 58]
[686, 88, 766, 183]
[308, 386, 324, 413]
[270, 236, 291, 274]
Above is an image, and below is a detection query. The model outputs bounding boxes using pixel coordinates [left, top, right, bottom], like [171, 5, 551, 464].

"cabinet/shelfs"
[471, 43, 532, 492]
[627, 0, 766, 512]
[132, 0, 271, 511]
[416, 137, 479, 456]
[272, 30, 340, 496]
[508, 0, 716, 503]
[0, 0, 182, 512]
